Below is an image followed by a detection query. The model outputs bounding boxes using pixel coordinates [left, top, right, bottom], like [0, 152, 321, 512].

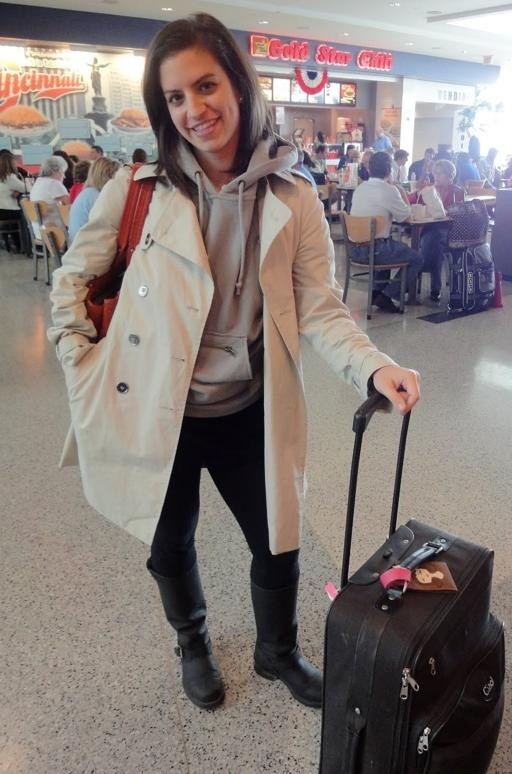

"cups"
[412, 203, 423, 221]
[24, 177, 34, 184]
[320, 152, 336, 158]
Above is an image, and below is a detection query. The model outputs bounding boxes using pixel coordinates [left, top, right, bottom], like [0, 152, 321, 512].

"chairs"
[39, 225, 66, 267]
[317, 183, 342, 240]
[56, 200, 72, 226]
[20, 198, 50, 285]
[338, 212, 410, 320]
[465, 178, 487, 195]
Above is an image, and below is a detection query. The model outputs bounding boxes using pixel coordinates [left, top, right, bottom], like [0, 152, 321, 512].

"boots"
[147, 558, 226, 706]
[253, 582, 327, 705]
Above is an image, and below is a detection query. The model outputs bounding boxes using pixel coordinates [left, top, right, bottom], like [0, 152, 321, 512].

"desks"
[391, 215, 452, 305]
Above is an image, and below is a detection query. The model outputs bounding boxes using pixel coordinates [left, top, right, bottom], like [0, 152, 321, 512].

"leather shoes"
[374, 296, 399, 313]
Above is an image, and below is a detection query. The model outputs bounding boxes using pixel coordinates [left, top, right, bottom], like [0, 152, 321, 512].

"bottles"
[411, 172, 416, 182]
[424, 172, 431, 185]
[338, 165, 345, 187]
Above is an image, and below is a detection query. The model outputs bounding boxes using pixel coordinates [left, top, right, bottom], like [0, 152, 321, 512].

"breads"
[0, 105, 51, 137]
[110, 108, 151, 128]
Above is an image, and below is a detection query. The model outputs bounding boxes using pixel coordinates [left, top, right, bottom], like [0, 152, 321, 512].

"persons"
[2, 141, 147, 262]
[86, 58, 110, 96]
[348, 152, 425, 313]
[338, 123, 410, 215]
[47, 9, 422, 711]
[407, 159, 465, 304]
[293, 129, 330, 214]
[407, 135, 510, 221]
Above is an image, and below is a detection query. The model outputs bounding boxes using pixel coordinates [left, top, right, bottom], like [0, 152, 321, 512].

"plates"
[110, 119, 153, 134]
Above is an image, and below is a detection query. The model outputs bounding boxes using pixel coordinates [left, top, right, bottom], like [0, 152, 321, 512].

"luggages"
[450, 244, 495, 311]
[318, 395, 505, 774]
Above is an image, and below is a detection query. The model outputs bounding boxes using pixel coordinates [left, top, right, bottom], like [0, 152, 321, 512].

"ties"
[397, 167, 402, 183]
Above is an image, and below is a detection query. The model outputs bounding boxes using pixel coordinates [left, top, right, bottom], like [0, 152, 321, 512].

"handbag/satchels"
[85, 269, 121, 336]
[448, 199, 489, 243]
